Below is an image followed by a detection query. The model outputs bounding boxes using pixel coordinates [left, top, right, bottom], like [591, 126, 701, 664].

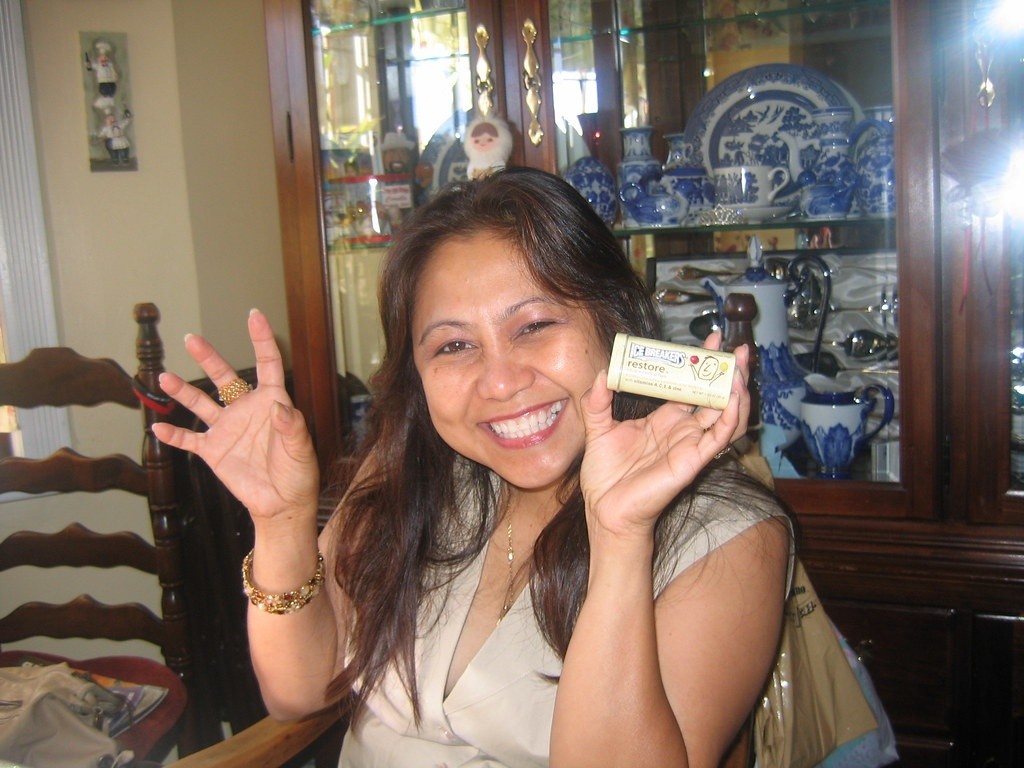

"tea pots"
[777, 107, 850, 220]
[618, 179, 695, 227]
[700, 235, 833, 478]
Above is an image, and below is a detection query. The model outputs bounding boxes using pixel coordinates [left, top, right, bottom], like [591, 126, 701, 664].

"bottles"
[617, 127, 662, 227]
[663, 133, 684, 228]
[850, 106, 894, 218]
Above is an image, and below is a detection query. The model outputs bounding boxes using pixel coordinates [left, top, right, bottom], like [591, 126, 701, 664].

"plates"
[682, 62, 868, 222]
[714, 206, 794, 224]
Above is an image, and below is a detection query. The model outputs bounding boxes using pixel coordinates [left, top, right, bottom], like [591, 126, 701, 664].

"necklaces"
[496, 481, 514, 625]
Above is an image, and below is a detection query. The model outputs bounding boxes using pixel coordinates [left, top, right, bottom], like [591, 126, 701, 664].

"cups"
[801, 374, 894, 479]
[714, 167, 789, 205]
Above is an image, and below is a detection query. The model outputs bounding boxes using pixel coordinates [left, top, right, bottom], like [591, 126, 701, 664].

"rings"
[704, 423, 730, 459]
[218, 378, 253, 405]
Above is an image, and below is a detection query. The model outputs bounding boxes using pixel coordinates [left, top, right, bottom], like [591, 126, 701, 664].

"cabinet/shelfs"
[264, 0, 1024, 768]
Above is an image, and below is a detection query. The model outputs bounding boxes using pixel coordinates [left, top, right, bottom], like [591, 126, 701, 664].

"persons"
[152, 166, 795, 768]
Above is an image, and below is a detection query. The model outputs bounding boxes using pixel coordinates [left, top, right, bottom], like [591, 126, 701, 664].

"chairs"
[0, 301, 228, 768]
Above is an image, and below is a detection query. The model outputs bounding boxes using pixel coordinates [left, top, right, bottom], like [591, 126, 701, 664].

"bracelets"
[242, 548, 323, 615]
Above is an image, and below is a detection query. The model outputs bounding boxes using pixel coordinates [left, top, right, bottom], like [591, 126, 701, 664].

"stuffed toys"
[464, 116, 513, 180]
